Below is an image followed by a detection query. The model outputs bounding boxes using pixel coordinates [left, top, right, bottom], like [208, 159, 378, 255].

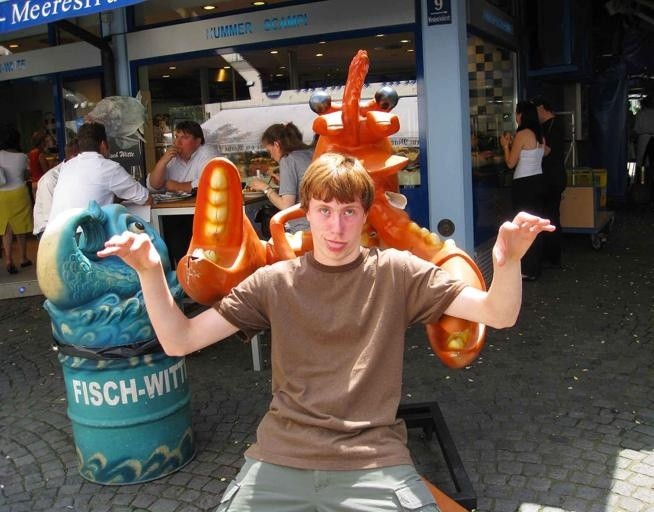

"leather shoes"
[19, 260, 33, 268]
[6, 263, 19, 275]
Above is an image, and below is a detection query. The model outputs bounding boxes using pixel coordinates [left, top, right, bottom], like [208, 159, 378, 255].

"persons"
[249, 122, 314, 241]
[501, 99, 567, 281]
[0, 122, 56, 274]
[32, 122, 153, 242]
[96, 152, 556, 512]
[153, 113, 169, 137]
[146, 121, 223, 195]
[626, 96, 654, 185]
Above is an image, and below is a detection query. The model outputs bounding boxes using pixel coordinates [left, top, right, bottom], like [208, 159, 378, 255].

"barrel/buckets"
[56, 347, 197, 486]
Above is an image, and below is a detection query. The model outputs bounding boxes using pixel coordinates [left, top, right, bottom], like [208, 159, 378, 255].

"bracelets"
[263, 186, 272, 195]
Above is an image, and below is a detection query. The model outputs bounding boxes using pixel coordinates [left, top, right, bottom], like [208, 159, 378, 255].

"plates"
[150, 191, 190, 202]
[242, 188, 265, 195]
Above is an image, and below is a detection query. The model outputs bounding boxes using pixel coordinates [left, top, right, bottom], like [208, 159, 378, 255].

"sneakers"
[521, 271, 538, 282]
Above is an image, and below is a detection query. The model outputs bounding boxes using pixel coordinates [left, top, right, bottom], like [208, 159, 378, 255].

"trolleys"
[560, 167, 616, 250]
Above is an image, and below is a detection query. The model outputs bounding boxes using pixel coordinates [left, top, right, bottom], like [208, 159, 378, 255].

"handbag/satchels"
[0, 166, 8, 187]
